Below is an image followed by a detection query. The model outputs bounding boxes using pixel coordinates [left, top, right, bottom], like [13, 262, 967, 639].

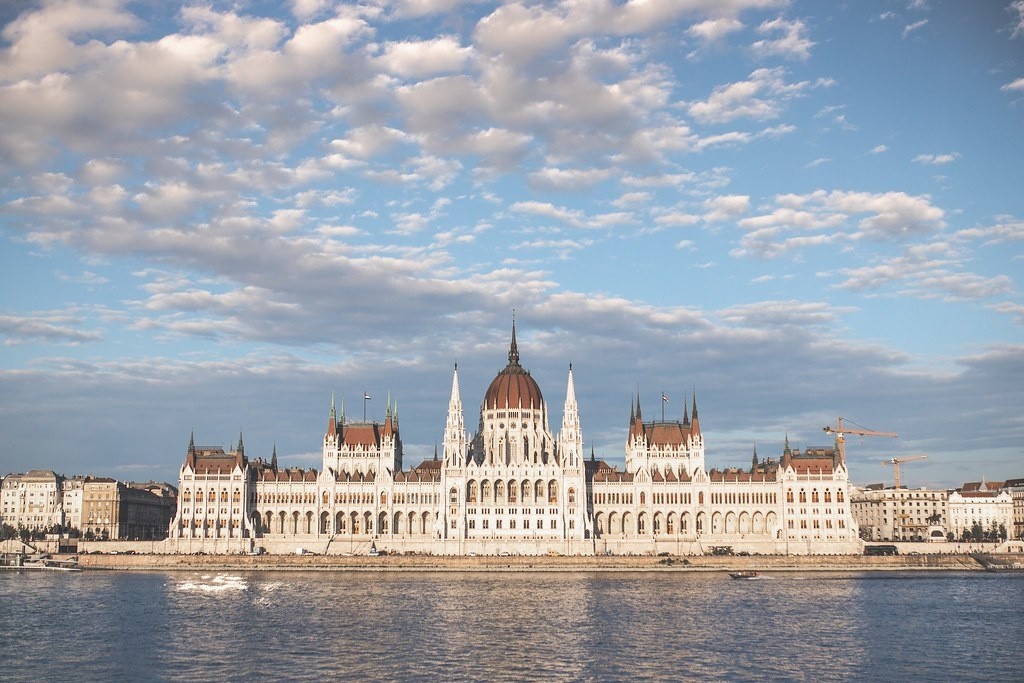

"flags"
[663, 395, 670, 403]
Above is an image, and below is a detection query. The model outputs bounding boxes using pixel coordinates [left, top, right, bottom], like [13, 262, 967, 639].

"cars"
[304, 553, 314, 556]
[125, 550, 135, 555]
[344, 552, 353, 557]
[501, 552, 510, 557]
[91, 551, 102, 555]
[467, 552, 475, 556]
[77, 551, 88, 554]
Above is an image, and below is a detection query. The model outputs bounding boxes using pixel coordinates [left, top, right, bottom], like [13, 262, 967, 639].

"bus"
[864, 545, 898, 556]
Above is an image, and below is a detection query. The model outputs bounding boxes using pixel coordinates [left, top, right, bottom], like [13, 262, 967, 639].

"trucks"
[253, 547, 265, 554]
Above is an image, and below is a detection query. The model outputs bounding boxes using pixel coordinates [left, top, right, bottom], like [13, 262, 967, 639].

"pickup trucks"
[110, 550, 122, 554]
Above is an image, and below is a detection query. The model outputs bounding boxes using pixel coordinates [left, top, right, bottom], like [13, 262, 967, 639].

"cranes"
[881, 455, 928, 490]
[823, 417, 898, 459]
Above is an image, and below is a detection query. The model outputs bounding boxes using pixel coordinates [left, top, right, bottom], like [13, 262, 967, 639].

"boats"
[729, 572, 760, 580]
[10, 558, 83, 570]
